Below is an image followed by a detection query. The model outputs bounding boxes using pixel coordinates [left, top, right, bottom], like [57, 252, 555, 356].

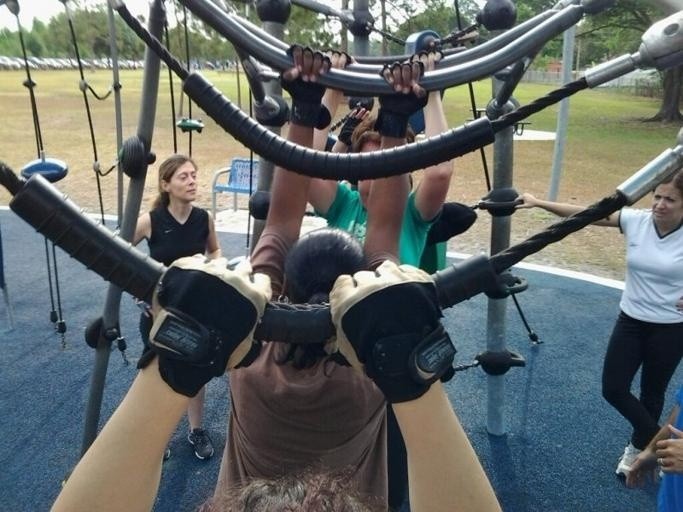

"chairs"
[212, 159, 259, 220]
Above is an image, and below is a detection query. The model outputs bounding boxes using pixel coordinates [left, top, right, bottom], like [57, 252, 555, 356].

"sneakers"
[186, 426, 215, 460]
[616, 438, 644, 478]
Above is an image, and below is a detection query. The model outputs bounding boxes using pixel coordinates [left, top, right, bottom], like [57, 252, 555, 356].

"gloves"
[279, 44, 331, 128]
[374, 62, 429, 140]
[323, 259, 457, 407]
[135, 250, 273, 399]
[339, 109, 376, 147]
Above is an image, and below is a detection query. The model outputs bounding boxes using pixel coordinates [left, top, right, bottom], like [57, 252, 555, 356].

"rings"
[658, 458, 664, 466]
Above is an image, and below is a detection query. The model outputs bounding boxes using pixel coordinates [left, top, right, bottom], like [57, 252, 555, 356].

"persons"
[43, 251, 502, 511]
[623, 381, 683, 512]
[111, 153, 215, 462]
[331, 107, 414, 192]
[202, 42, 430, 512]
[305, 48, 455, 511]
[516, 170, 682, 480]
